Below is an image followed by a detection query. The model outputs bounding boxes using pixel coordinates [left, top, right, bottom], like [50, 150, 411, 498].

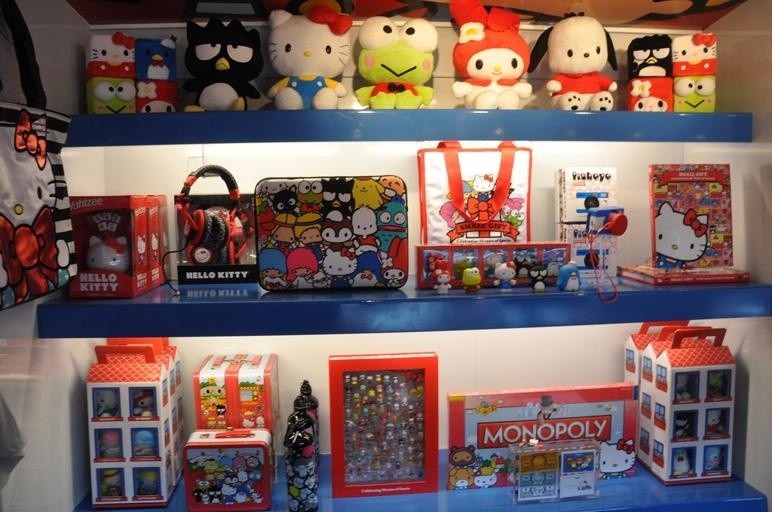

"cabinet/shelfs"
[36, 111, 772, 512]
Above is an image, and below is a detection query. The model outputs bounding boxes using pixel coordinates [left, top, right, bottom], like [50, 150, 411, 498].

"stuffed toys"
[430, 259, 455, 296]
[353, 14, 439, 112]
[446, 0, 534, 113]
[172, 16, 265, 114]
[84, 75, 138, 118]
[672, 369, 730, 475]
[622, 34, 674, 79]
[525, 260, 550, 291]
[556, 264, 581, 292]
[622, 74, 674, 117]
[524, 10, 618, 115]
[493, 260, 516, 292]
[95, 388, 163, 499]
[133, 79, 179, 116]
[461, 266, 484, 295]
[84, 31, 138, 78]
[666, 32, 720, 78]
[135, 35, 178, 82]
[671, 73, 719, 114]
[260, 4, 355, 111]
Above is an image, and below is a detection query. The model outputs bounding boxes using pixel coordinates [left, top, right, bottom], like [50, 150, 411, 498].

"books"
[646, 161, 735, 269]
[615, 258, 752, 287]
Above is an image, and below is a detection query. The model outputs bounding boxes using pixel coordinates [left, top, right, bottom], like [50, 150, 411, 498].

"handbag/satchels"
[418, 140, 532, 244]
[0, 99, 78, 311]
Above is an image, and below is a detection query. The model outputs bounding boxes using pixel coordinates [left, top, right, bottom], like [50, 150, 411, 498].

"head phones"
[173, 163, 253, 265]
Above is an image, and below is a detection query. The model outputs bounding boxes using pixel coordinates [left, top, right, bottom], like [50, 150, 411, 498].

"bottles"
[284, 379, 320, 512]
